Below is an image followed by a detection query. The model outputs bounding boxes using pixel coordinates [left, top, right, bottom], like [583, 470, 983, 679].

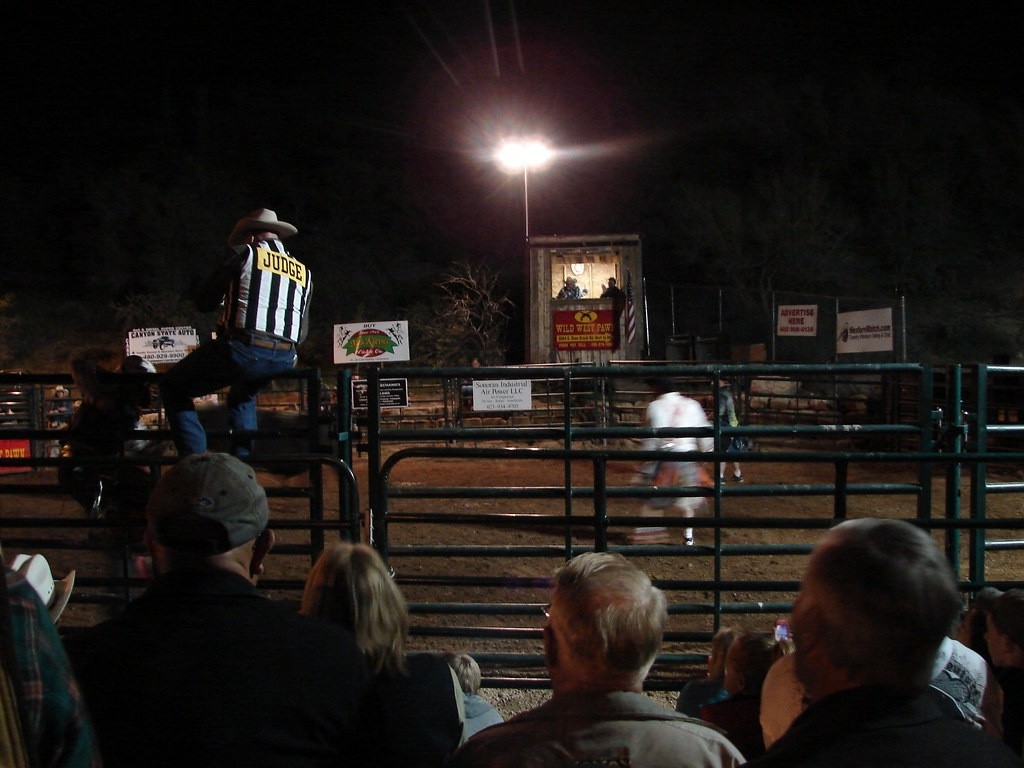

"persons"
[57, 209, 313, 518]
[556, 278, 579, 299]
[447, 551, 747, 768]
[674, 516, 1024, 768]
[600, 278, 626, 299]
[47, 385, 73, 429]
[626, 373, 742, 546]
[0, 452, 504, 768]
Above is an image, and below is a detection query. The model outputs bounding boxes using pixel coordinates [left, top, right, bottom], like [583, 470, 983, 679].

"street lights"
[495, 134, 551, 241]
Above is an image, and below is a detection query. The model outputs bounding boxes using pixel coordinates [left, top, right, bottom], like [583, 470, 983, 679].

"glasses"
[540, 603, 553, 619]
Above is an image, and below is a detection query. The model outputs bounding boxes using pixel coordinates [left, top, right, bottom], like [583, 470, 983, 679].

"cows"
[201, 405, 347, 478]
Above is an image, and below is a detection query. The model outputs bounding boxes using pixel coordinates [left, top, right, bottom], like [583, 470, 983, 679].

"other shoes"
[720, 477, 726, 485]
[731, 475, 744, 483]
[682, 536, 694, 546]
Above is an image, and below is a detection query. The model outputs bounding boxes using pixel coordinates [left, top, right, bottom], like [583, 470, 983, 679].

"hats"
[984, 588, 1024, 651]
[123, 355, 158, 385]
[51, 385, 69, 397]
[710, 379, 731, 390]
[227, 208, 299, 251]
[145, 451, 272, 558]
[8, 552, 76, 625]
[563, 277, 577, 284]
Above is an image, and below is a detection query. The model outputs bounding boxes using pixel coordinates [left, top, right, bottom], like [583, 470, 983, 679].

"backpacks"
[628, 440, 673, 476]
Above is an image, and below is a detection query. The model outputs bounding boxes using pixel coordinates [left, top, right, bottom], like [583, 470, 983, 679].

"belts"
[223, 330, 291, 351]
